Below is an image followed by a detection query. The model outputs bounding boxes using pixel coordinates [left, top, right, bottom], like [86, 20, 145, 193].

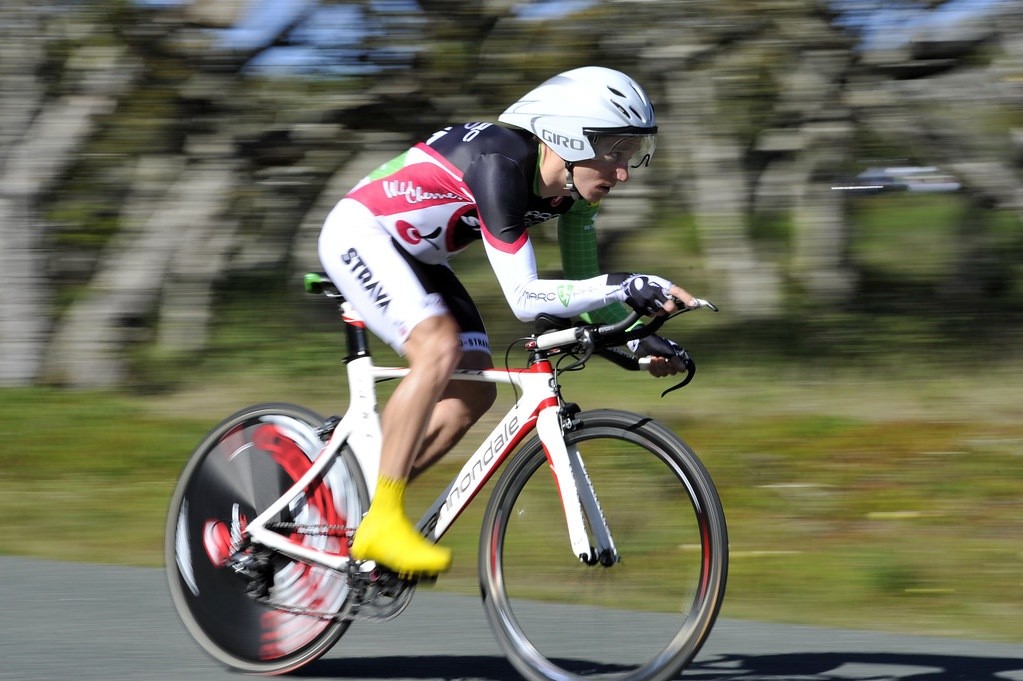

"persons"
[318, 67, 698, 569]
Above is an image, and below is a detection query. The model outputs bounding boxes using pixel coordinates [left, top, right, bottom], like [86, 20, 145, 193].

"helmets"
[497, 66, 658, 168]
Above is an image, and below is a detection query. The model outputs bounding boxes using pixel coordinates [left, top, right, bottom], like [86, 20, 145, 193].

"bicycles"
[163, 272, 729, 681]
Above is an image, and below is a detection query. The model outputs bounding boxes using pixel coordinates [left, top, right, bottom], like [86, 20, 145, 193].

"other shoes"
[351, 477, 454, 573]
